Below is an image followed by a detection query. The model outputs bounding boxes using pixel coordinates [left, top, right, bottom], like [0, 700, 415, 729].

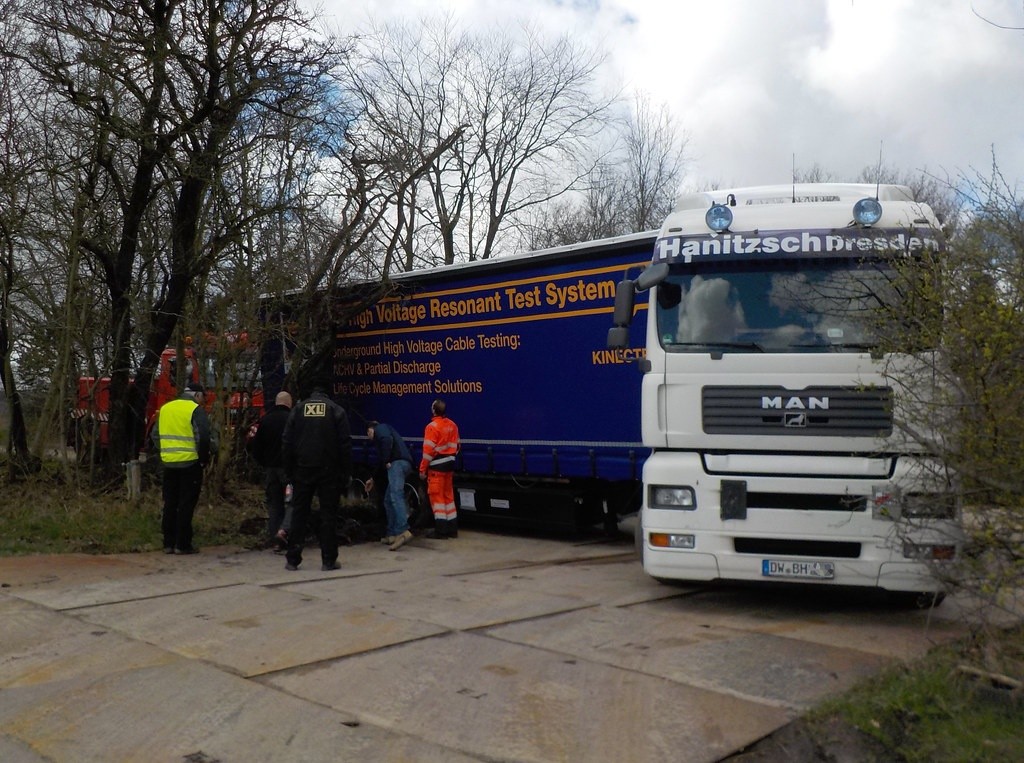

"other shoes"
[273, 534, 288, 551]
[381, 535, 395, 543]
[285, 563, 297, 571]
[164, 546, 200, 554]
[427, 531, 458, 539]
[321, 561, 342, 571]
[388, 530, 414, 550]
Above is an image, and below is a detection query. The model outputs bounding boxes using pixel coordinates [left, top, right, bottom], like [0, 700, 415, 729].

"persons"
[158, 383, 209, 554]
[247, 380, 351, 573]
[365, 420, 414, 551]
[418, 399, 460, 538]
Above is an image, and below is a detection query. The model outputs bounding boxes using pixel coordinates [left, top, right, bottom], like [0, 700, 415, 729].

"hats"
[184, 383, 208, 396]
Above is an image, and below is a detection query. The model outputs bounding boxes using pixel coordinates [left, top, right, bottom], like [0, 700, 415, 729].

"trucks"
[69, 330, 265, 474]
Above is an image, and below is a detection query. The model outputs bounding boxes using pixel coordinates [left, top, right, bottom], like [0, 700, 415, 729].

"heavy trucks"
[262, 182, 984, 610]
